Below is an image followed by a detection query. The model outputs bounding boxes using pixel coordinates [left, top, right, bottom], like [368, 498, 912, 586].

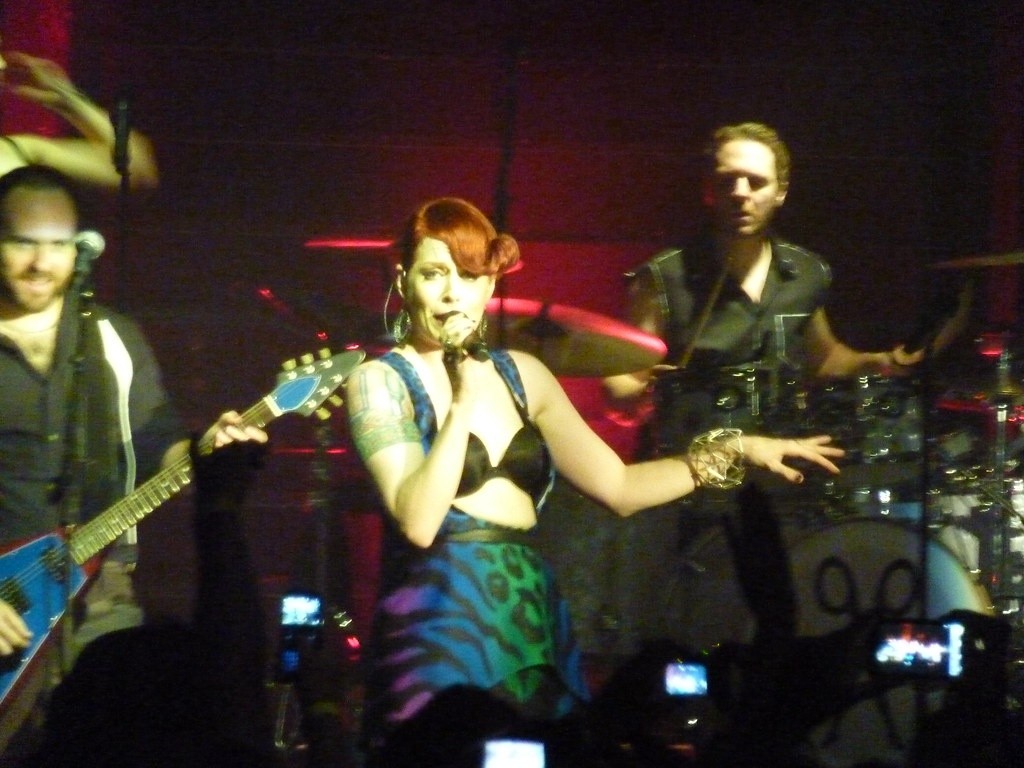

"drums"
[650, 354, 796, 506]
[789, 370, 943, 492]
[662, 513, 996, 768]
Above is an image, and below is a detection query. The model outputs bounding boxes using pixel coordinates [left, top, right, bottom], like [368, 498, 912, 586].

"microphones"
[443, 311, 490, 362]
[74, 230, 105, 286]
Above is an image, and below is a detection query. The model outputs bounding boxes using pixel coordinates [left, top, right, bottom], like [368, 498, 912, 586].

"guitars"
[0, 344, 369, 720]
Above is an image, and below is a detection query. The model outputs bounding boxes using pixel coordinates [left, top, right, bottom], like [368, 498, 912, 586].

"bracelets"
[686, 426, 746, 489]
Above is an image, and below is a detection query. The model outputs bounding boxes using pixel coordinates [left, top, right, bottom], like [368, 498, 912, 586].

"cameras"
[281, 596, 324, 628]
[483, 739, 546, 768]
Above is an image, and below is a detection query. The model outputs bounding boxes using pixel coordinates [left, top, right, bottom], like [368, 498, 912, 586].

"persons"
[1, 50, 162, 192]
[346, 199, 844, 767]
[33, 623, 264, 768]
[0, 164, 270, 768]
[601, 121, 924, 702]
[378, 685, 552, 768]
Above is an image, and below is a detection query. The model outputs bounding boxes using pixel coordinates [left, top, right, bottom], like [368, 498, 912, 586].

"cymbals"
[478, 296, 669, 381]
[927, 249, 1024, 272]
[917, 331, 1023, 413]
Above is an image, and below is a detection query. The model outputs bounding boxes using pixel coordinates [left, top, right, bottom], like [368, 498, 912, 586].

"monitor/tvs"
[665, 663, 708, 696]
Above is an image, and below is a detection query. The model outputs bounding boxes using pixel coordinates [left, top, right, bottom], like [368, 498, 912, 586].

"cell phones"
[873, 617, 974, 678]
